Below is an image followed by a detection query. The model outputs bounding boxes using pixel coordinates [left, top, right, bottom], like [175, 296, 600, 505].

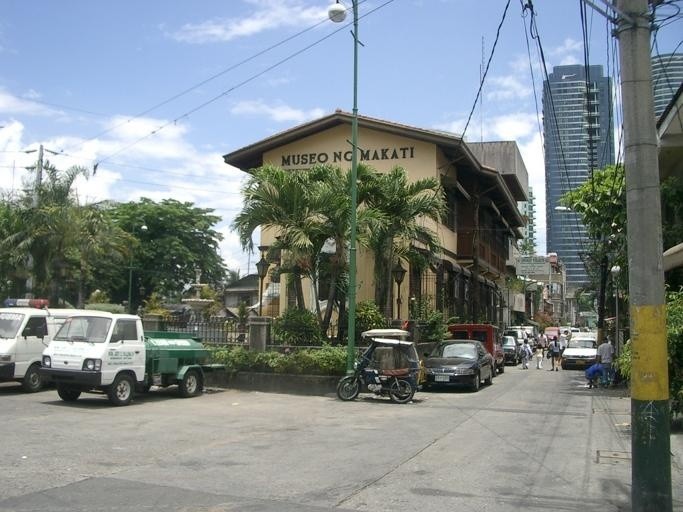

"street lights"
[328, 0, 364, 376]
[255, 245, 271, 316]
[611, 261, 622, 357]
[127, 217, 148, 314]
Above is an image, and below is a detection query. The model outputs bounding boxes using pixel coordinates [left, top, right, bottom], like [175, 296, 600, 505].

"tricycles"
[335, 328, 423, 404]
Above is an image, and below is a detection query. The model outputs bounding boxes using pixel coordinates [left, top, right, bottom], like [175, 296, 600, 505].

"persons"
[518, 339, 533, 369]
[585, 363, 605, 389]
[558, 330, 570, 366]
[548, 335, 561, 371]
[597, 337, 615, 388]
[535, 343, 544, 369]
[537, 330, 547, 361]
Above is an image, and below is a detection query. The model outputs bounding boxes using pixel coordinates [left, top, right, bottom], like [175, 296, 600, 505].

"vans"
[0, 297, 118, 394]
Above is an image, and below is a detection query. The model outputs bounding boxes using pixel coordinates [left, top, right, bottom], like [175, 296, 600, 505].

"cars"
[420, 323, 538, 392]
[542, 324, 598, 370]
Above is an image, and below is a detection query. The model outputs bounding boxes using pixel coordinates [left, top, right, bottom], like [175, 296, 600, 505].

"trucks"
[37, 312, 212, 407]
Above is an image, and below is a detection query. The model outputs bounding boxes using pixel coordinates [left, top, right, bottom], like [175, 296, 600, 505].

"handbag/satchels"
[521, 350, 527, 358]
